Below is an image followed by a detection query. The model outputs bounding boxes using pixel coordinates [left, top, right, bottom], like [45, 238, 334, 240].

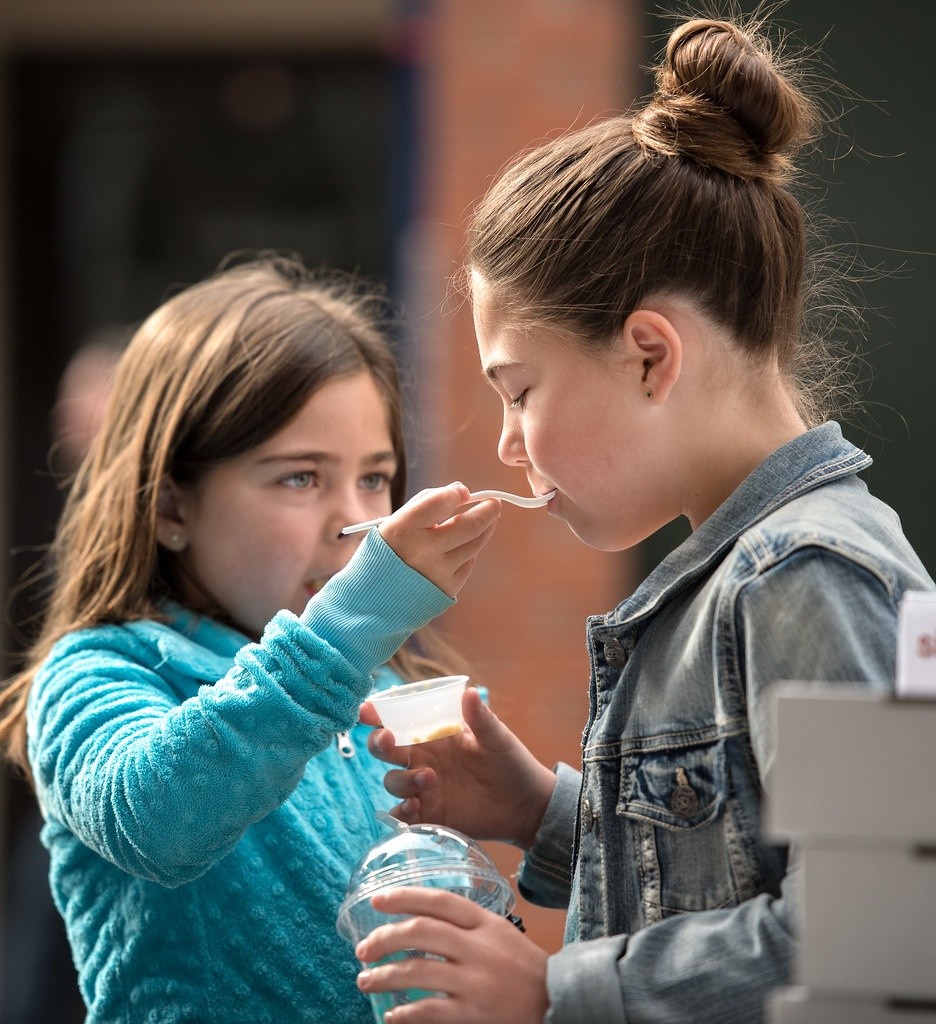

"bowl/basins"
[365, 674, 469, 747]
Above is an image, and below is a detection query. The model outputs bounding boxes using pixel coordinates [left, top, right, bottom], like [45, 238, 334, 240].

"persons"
[0, 263, 502, 1024]
[354, 18, 936, 1024]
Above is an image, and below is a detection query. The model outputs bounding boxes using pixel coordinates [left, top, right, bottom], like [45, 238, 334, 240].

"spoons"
[341, 489, 556, 534]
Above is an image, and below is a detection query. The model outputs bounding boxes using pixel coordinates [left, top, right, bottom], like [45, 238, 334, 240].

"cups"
[336, 824, 517, 1024]
